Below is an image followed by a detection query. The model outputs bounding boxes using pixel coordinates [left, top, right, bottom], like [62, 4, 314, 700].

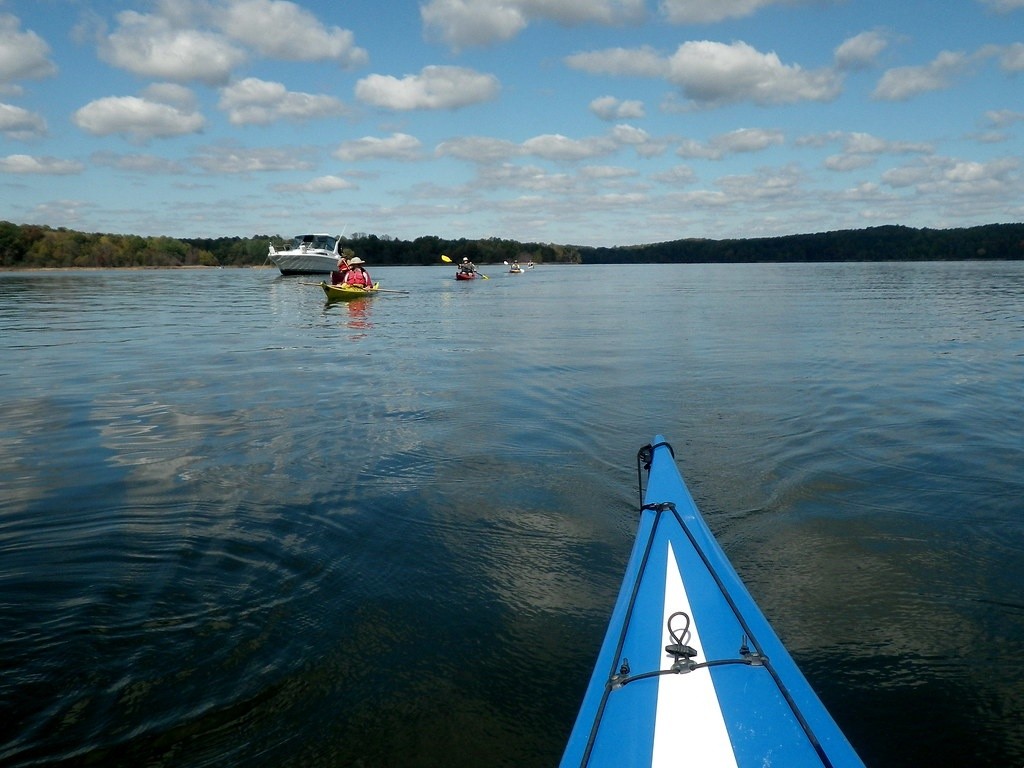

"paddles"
[342, 260, 351, 271]
[297, 281, 341, 288]
[469, 268, 489, 279]
[441, 255, 464, 268]
[518, 268, 524, 273]
[369, 289, 410, 294]
[504, 261, 513, 266]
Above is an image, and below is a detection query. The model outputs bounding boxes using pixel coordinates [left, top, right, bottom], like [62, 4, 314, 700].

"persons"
[337, 255, 351, 272]
[512, 260, 519, 269]
[458, 257, 476, 277]
[343, 256, 372, 290]
[528, 260, 534, 265]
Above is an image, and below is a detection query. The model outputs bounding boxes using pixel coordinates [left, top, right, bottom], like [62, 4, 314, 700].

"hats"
[463, 257, 468, 260]
[346, 260, 352, 266]
[351, 256, 365, 264]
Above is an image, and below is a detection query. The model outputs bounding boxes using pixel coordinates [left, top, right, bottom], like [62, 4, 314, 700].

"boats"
[509, 270, 522, 272]
[455, 272, 476, 281]
[268, 233, 344, 277]
[319, 280, 378, 299]
[527, 266, 534, 269]
